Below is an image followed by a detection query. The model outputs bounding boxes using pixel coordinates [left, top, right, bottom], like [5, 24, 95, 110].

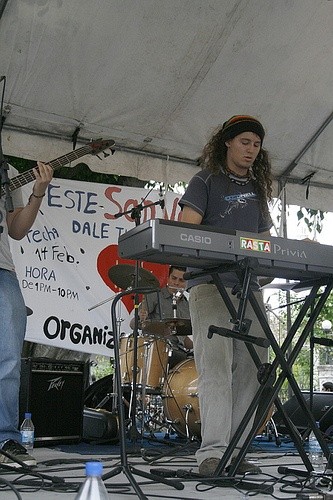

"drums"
[117, 334, 275, 440]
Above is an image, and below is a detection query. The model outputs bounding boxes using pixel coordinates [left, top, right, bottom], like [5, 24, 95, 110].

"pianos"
[118, 218, 333, 491]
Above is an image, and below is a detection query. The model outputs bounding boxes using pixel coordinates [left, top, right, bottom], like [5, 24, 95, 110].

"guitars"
[0, 137, 117, 201]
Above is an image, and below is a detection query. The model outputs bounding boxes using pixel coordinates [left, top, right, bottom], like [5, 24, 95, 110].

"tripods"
[95, 286, 184, 500]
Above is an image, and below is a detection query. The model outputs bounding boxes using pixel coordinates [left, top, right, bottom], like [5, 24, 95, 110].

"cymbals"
[107, 264, 162, 294]
[141, 317, 193, 337]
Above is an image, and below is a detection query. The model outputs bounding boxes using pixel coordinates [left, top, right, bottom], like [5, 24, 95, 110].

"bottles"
[73, 462, 111, 500]
[18, 413, 34, 454]
[308, 421, 324, 472]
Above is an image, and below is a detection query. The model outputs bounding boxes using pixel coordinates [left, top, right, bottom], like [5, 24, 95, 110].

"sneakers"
[225, 457, 261, 475]
[0, 440, 37, 468]
[199, 458, 221, 477]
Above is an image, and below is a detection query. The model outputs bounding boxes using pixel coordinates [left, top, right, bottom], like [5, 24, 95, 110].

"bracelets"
[29, 193, 45, 205]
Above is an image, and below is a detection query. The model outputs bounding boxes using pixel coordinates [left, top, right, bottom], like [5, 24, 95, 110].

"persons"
[179, 115, 274, 477]
[130, 265, 193, 369]
[0, 162, 53, 468]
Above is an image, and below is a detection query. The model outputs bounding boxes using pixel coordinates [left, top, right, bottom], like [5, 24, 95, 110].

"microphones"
[159, 186, 167, 209]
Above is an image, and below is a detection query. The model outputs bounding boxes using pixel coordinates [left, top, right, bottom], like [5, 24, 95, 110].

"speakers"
[20, 358, 88, 448]
[274, 390, 333, 440]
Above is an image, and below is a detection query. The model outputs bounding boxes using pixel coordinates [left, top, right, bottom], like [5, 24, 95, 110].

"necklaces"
[225, 165, 249, 179]
